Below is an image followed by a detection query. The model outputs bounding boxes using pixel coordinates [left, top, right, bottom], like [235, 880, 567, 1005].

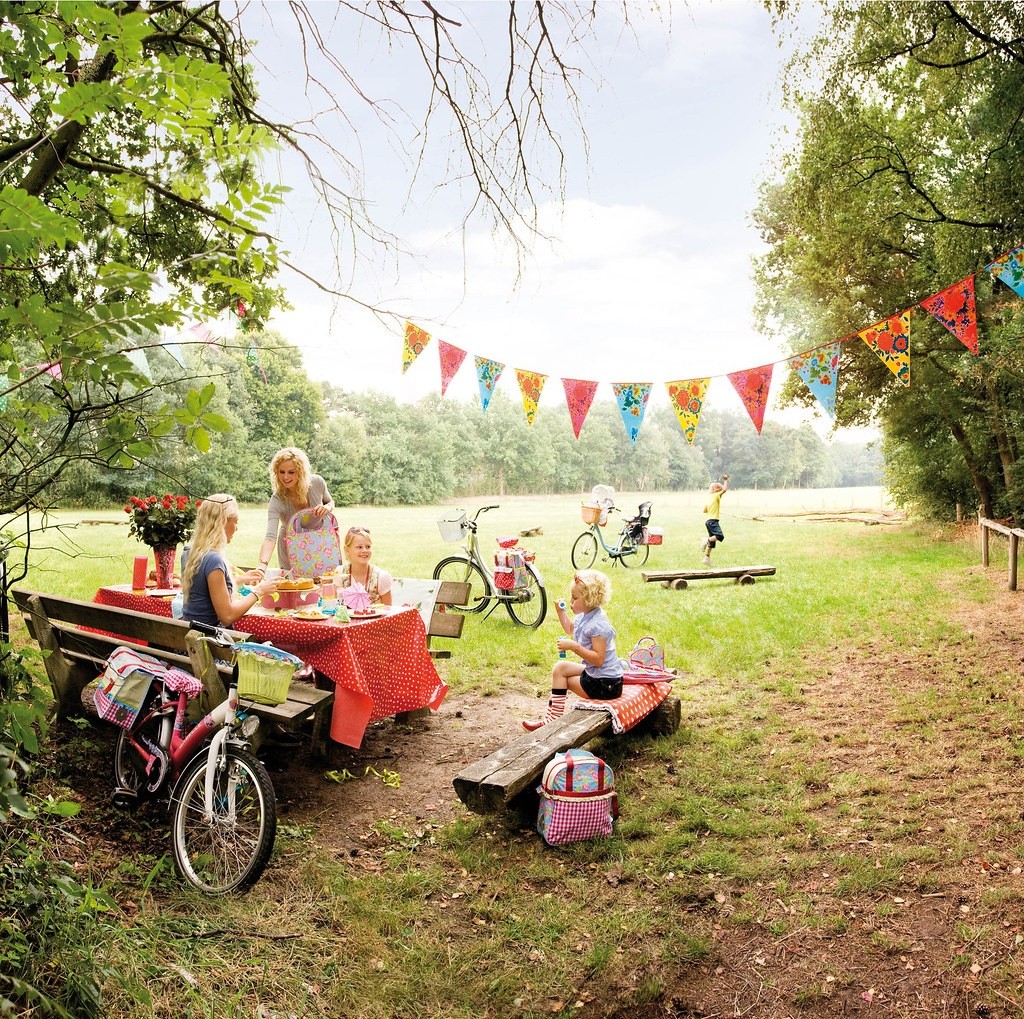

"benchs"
[452, 667, 681, 831]
[641, 565, 777, 590]
[12, 587, 335, 764]
[235, 565, 472, 726]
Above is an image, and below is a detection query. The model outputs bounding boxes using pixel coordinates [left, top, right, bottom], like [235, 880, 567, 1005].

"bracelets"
[250, 591, 260, 601]
[574, 644, 579, 655]
[257, 558, 268, 567]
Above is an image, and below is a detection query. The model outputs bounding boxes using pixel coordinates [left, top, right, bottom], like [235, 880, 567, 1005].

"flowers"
[125, 492, 203, 580]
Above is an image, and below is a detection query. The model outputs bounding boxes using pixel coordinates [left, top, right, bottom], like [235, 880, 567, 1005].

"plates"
[292, 614, 329, 619]
[348, 609, 387, 617]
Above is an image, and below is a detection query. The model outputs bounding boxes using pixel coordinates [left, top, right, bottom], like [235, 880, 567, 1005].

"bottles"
[558, 636, 566, 659]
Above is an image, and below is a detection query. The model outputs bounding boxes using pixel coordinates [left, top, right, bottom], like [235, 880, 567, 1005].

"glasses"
[347, 527, 370, 539]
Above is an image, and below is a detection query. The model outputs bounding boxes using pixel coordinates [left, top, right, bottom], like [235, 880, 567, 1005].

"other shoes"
[521, 720, 543, 733]
[702, 557, 715, 567]
[700, 537, 710, 552]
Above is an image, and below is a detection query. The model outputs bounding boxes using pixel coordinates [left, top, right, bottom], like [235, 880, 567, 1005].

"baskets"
[582, 507, 602, 524]
[237, 651, 297, 705]
[437, 509, 467, 542]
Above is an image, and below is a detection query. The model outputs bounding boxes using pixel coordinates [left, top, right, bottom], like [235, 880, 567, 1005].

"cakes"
[275, 577, 315, 590]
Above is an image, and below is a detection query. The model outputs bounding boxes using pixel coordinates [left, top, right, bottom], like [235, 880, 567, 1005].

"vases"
[152, 543, 176, 590]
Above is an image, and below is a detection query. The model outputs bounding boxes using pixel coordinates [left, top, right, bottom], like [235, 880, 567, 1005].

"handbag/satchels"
[93, 646, 155, 731]
[642, 527, 662, 545]
[536, 748, 619, 846]
[630, 637, 664, 670]
[493, 549, 528, 590]
[286, 508, 342, 578]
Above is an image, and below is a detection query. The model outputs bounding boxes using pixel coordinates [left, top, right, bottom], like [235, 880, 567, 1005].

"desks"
[82, 588, 450, 765]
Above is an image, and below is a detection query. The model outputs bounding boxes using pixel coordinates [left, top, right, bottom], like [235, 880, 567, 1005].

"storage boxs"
[641, 526, 664, 545]
[493, 566, 529, 590]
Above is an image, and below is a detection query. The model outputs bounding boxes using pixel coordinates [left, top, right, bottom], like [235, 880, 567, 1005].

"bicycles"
[570, 498, 656, 570]
[110, 620, 304, 898]
[433, 504, 547, 629]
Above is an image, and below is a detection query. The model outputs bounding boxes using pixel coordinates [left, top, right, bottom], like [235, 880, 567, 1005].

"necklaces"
[349, 562, 370, 593]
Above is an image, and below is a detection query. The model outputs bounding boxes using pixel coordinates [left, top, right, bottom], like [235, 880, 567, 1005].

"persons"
[250, 448, 342, 594]
[330, 527, 392, 607]
[522, 569, 623, 734]
[700, 475, 729, 567]
[164, 493, 285, 666]
